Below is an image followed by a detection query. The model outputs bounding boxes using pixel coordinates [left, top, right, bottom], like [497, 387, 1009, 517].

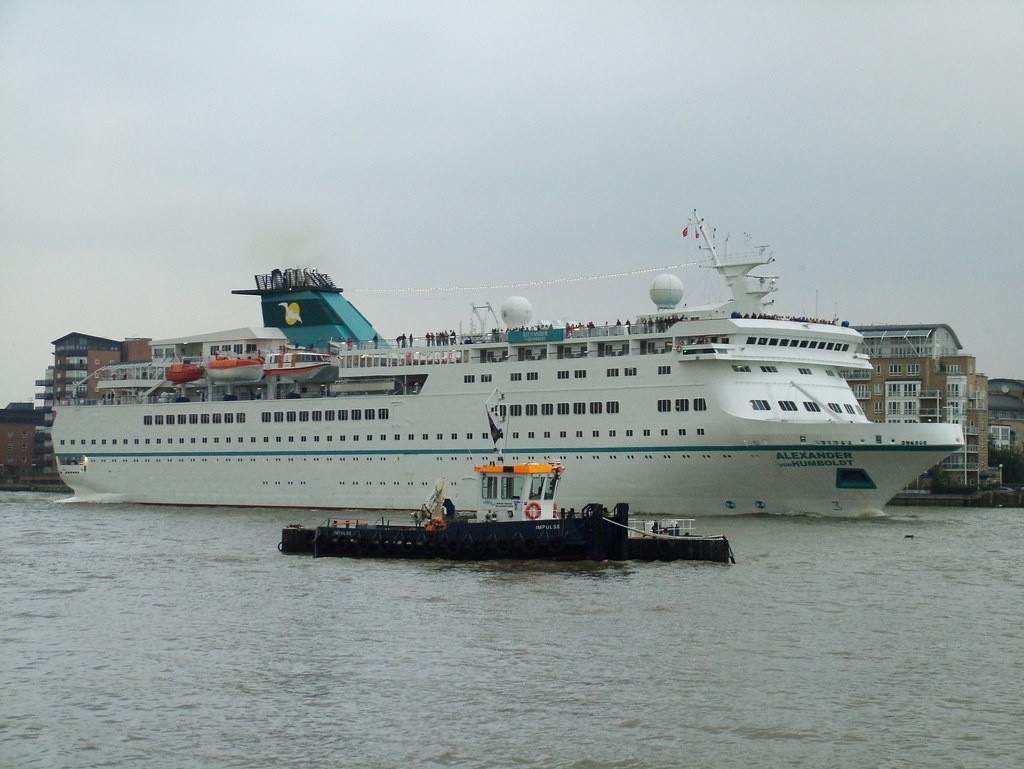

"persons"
[512, 324, 553, 331]
[644, 315, 684, 333]
[426, 330, 456, 346]
[566, 322, 584, 338]
[617, 318, 621, 325]
[587, 321, 595, 329]
[409, 334, 414, 347]
[396, 333, 406, 348]
[736, 312, 835, 325]
[290, 379, 302, 399]
[626, 320, 630, 334]
[605, 322, 608, 336]
[492, 327, 512, 342]
[253, 385, 262, 400]
[373, 334, 378, 349]
[691, 337, 709, 344]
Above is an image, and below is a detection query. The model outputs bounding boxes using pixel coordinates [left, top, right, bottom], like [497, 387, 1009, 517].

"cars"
[985, 486, 1014, 492]
[1017, 485, 1024, 492]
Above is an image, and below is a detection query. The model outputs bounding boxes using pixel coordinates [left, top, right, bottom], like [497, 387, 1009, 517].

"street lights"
[998, 464, 1003, 486]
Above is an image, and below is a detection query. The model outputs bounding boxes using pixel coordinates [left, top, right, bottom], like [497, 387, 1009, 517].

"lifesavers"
[525, 503, 542, 519]
[676, 346, 682, 352]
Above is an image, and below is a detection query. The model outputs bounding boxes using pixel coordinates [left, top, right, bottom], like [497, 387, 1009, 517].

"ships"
[48, 205, 966, 520]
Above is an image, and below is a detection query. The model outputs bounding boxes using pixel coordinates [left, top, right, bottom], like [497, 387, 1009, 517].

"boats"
[164, 360, 205, 383]
[264, 345, 341, 386]
[278, 386, 738, 566]
[206, 354, 266, 380]
